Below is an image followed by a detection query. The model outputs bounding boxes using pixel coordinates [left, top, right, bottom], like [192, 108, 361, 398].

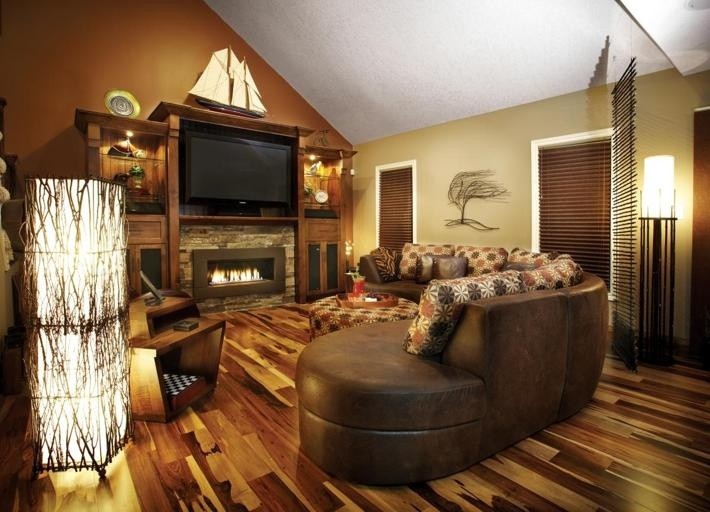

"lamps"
[23, 178, 134, 481]
[642, 155, 675, 217]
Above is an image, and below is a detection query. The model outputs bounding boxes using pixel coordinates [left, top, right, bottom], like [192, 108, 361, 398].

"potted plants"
[345, 266, 366, 298]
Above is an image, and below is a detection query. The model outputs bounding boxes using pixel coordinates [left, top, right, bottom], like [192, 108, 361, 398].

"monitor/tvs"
[185, 130, 292, 217]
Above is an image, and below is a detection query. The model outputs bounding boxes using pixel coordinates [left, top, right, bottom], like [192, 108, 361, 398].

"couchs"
[295, 253, 608, 487]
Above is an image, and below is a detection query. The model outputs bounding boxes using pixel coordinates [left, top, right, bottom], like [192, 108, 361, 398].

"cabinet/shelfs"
[0, 153, 24, 355]
[298, 144, 346, 304]
[74, 107, 168, 295]
[126, 290, 225, 423]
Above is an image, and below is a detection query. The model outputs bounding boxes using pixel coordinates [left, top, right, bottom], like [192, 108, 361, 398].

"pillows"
[454, 246, 507, 276]
[552, 254, 584, 284]
[521, 262, 574, 291]
[371, 246, 403, 282]
[505, 247, 555, 270]
[398, 243, 456, 280]
[401, 270, 522, 356]
[415, 255, 448, 283]
[433, 256, 468, 279]
